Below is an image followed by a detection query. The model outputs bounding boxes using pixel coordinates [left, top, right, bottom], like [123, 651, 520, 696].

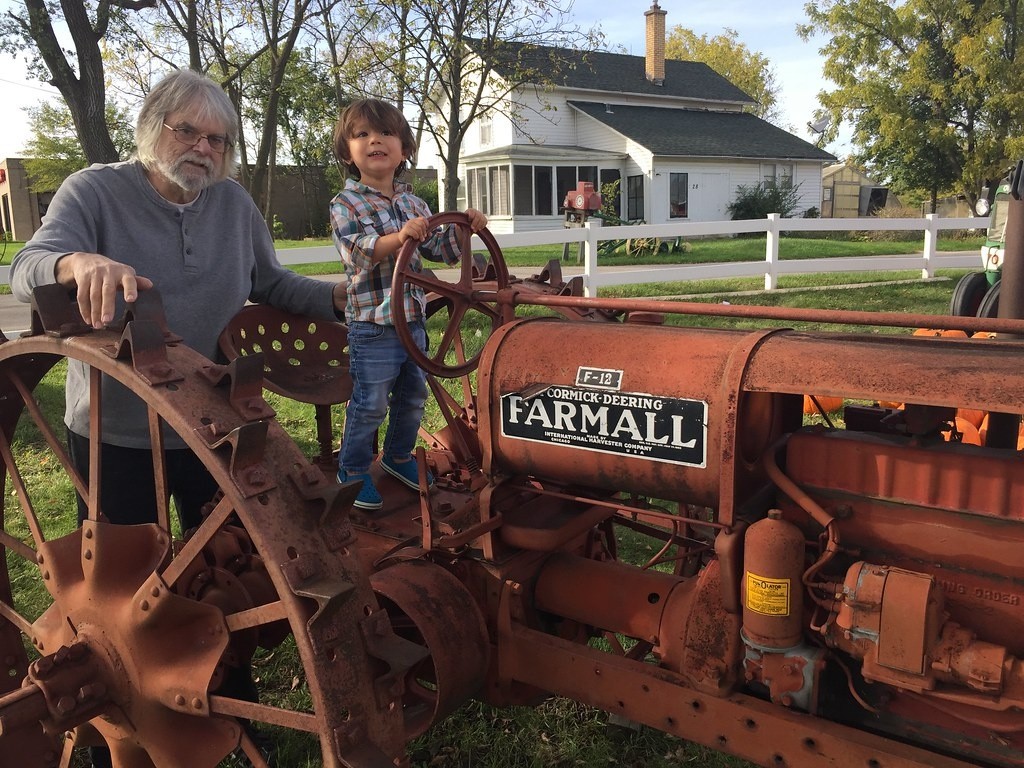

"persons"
[10, 71, 351, 768]
[330, 98, 488, 511]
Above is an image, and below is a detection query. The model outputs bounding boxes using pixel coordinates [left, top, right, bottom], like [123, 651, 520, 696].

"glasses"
[163, 122, 234, 155]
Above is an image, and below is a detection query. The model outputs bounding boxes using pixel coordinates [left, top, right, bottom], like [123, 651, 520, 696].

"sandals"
[335, 465, 384, 511]
[377, 453, 435, 493]
